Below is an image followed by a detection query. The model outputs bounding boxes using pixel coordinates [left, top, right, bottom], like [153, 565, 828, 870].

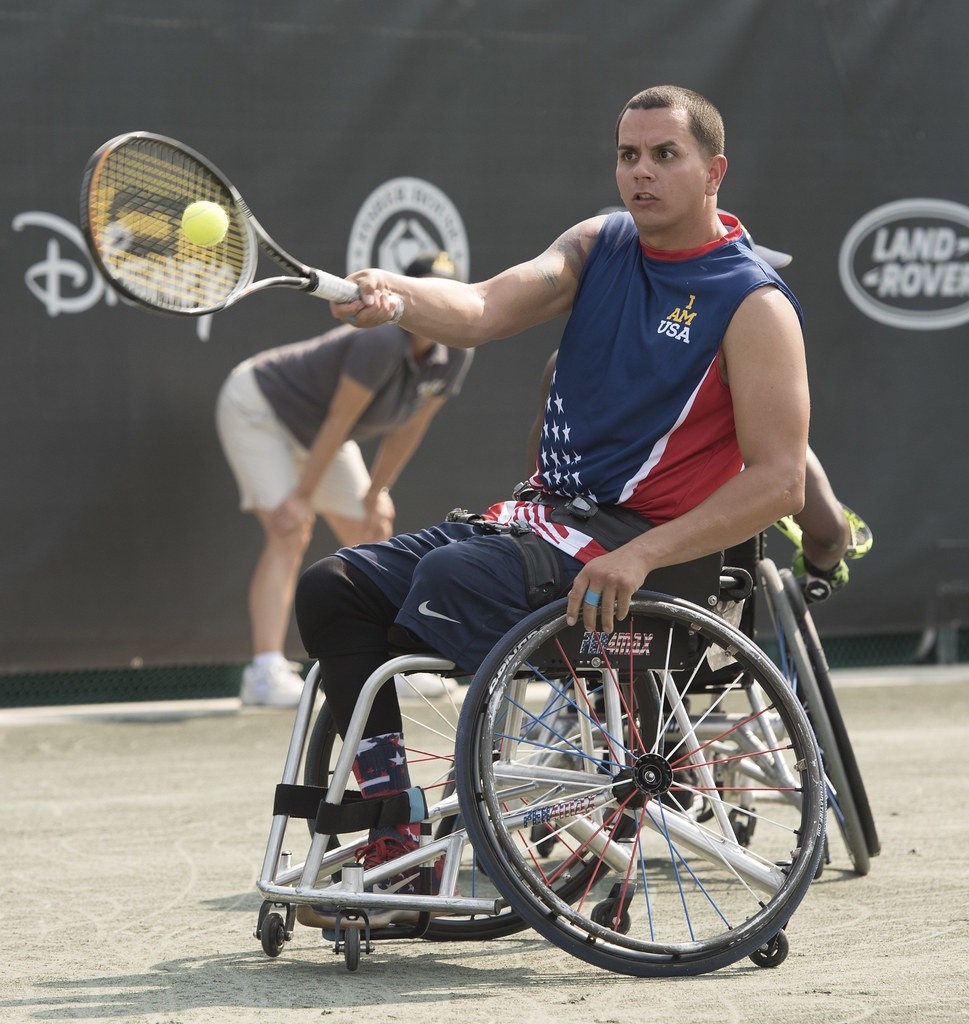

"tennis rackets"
[774, 501, 875, 604]
[76, 128, 406, 325]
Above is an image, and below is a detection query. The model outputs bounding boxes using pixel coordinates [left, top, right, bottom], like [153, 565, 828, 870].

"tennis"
[180, 198, 233, 248]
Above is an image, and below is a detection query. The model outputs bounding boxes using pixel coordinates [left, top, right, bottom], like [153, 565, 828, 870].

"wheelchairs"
[252, 526, 881, 977]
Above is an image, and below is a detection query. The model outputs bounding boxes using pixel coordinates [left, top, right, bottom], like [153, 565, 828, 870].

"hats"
[405, 252, 459, 281]
[716, 208, 793, 269]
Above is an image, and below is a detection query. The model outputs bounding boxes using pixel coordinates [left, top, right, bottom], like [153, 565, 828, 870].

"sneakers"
[297, 827, 462, 930]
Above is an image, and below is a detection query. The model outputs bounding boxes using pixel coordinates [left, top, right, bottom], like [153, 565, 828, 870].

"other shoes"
[240, 661, 325, 706]
[394, 674, 458, 698]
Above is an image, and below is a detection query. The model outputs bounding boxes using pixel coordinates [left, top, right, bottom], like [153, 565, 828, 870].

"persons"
[295, 86, 850, 914]
[215, 253, 475, 708]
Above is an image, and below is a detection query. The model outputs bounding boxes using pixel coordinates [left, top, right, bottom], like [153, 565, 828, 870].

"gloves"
[790, 549, 849, 604]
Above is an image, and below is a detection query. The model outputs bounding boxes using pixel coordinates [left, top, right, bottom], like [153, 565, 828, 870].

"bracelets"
[803, 555, 841, 581]
